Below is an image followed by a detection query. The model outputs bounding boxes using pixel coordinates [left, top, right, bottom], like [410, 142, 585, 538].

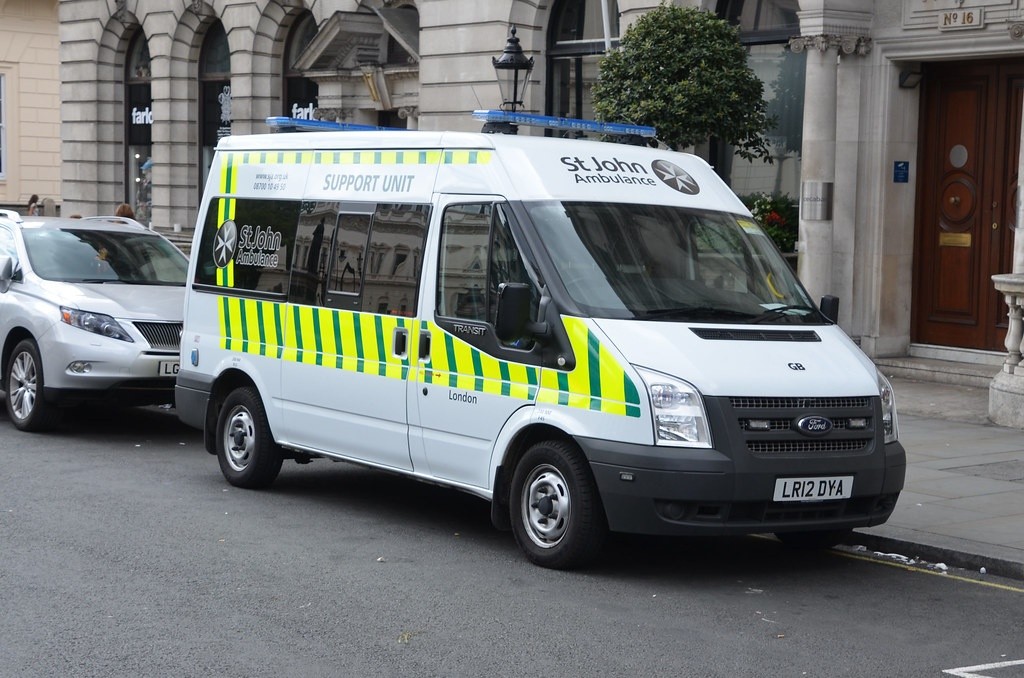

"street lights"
[491, 24, 535, 135]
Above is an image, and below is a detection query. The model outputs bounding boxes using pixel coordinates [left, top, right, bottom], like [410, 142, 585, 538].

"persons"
[28, 194, 39, 216]
[116, 204, 135, 220]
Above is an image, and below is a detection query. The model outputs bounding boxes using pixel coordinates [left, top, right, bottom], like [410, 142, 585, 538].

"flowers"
[694, 191, 799, 254]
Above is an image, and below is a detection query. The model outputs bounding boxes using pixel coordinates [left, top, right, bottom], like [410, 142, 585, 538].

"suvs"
[0, 209, 189, 431]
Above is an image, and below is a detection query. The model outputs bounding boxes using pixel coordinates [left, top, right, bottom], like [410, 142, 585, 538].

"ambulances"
[176, 108, 907, 573]
